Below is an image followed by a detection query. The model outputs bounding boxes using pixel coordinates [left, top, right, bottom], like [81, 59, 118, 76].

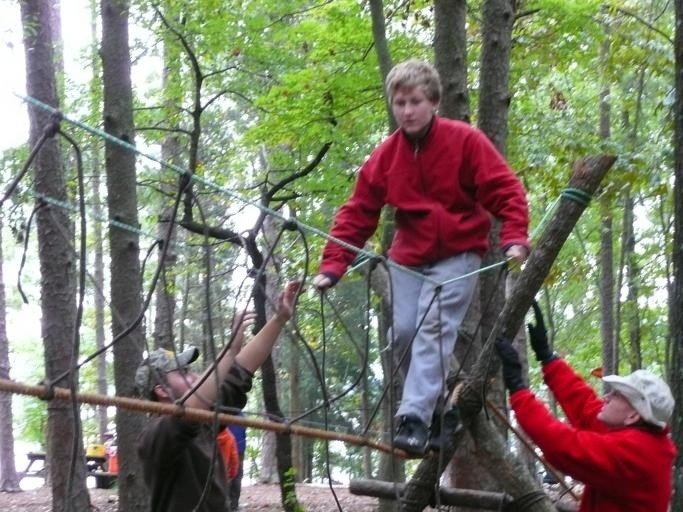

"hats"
[135, 347, 199, 400]
[601, 368, 678, 432]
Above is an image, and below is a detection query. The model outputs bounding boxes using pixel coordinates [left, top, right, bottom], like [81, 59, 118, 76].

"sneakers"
[391, 410, 467, 456]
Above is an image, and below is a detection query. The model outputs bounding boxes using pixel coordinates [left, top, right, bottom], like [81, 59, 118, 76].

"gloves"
[494, 338, 527, 395]
[528, 300, 549, 362]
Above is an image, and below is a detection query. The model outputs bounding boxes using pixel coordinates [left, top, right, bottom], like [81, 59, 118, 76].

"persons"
[216, 427, 239, 485]
[132, 280, 309, 511]
[494, 296, 679, 511]
[226, 412, 246, 511]
[306, 58, 535, 460]
